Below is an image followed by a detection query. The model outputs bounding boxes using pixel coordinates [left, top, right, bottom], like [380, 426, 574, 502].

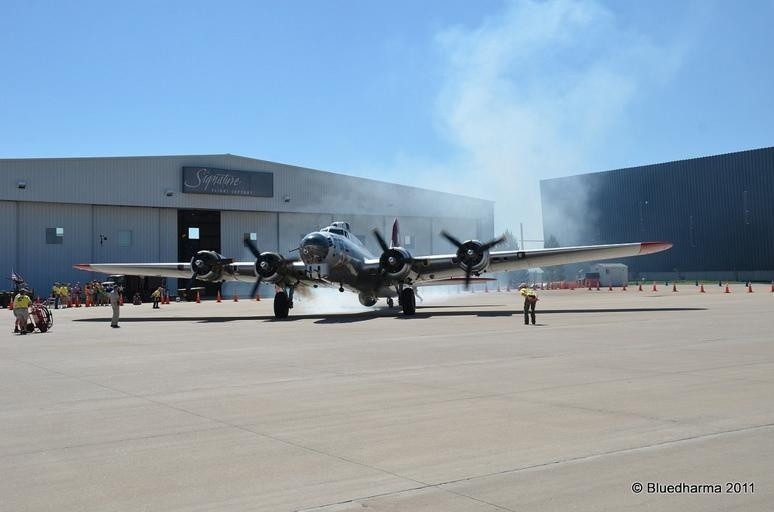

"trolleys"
[27, 303, 54, 333]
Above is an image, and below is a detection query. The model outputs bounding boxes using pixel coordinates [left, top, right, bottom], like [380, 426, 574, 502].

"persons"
[14, 288, 32, 334]
[132, 293, 142, 305]
[11, 288, 27, 333]
[108, 285, 121, 328]
[151, 286, 162, 309]
[517, 283, 539, 324]
[51, 280, 112, 309]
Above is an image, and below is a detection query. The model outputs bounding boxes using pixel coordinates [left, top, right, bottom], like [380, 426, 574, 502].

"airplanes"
[73, 217, 674, 319]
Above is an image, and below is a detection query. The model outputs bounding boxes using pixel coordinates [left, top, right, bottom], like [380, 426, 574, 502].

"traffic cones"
[67, 290, 263, 307]
